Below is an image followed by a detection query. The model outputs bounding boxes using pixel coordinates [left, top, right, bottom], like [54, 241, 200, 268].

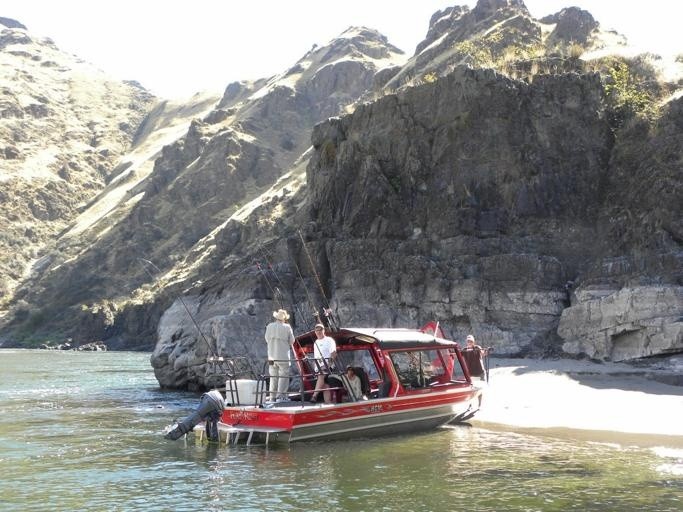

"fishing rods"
[137, 257, 236, 381]
[254, 227, 341, 375]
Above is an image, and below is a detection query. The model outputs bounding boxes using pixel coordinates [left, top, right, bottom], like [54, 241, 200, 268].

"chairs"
[354, 366, 380, 399]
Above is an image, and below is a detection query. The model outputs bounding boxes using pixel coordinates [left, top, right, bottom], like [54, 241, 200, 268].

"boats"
[166, 328, 487, 447]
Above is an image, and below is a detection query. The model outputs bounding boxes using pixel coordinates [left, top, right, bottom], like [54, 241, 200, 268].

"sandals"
[310, 395, 317, 402]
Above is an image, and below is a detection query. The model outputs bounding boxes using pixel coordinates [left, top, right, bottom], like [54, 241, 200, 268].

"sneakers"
[276, 398, 291, 403]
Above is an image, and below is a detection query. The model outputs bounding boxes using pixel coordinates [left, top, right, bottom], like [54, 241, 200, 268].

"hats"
[273, 309, 290, 322]
[314, 323, 325, 329]
[466, 334, 474, 343]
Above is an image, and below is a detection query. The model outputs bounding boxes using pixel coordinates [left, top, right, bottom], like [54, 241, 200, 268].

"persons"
[265, 309, 295, 403]
[308, 324, 337, 403]
[344, 367, 362, 399]
[459, 335, 489, 411]
